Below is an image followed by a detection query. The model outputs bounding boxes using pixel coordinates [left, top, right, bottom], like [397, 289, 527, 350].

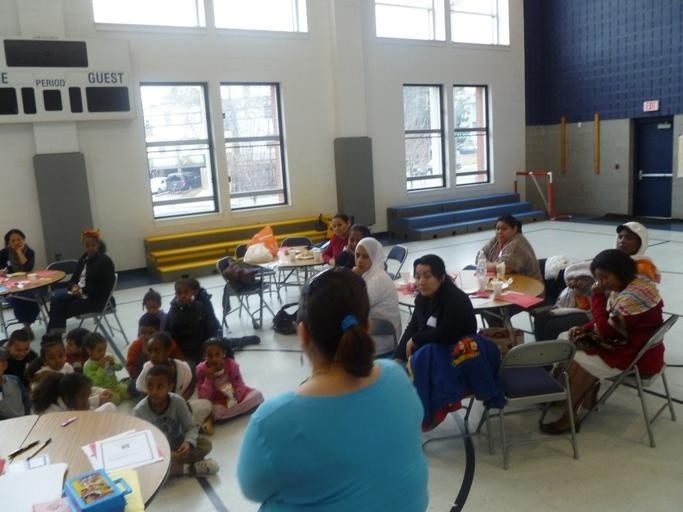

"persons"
[237, 265, 429, 511]
[127, 277, 263, 479]
[316, 213, 351, 266]
[474, 217, 547, 328]
[0, 327, 131, 423]
[0, 229, 42, 340]
[392, 254, 478, 367]
[336, 226, 402, 354]
[46, 231, 116, 332]
[533, 221, 665, 433]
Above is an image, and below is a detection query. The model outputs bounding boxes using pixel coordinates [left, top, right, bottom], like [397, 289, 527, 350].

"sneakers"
[202, 417, 215, 435]
[194, 459, 219, 476]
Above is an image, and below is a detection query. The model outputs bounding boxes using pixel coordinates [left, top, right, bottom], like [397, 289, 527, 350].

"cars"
[454, 143, 477, 155]
[410, 154, 462, 176]
[148, 177, 167, 193]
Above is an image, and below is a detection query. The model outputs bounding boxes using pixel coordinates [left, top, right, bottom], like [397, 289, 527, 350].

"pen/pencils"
[8, 441, 39, 457]
[27, 438, 52, 461]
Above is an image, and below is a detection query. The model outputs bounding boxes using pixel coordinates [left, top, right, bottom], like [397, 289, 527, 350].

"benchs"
[140, 215, 331, 279]
[386, 194, 544, 237]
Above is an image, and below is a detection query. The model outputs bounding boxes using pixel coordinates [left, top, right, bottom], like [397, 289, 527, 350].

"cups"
[28, 273, 39, 281]
[312, 251, 320, 261]
[492, 281, 502, 296]
[477, 277, 488, 290]
[289, 248, 295, 260]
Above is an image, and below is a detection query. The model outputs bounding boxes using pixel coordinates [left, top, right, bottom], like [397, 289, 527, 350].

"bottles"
[477, 251, 486, 277]
[496, 254, 505, 279]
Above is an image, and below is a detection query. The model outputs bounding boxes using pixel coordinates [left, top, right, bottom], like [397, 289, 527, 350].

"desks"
[393, 269, 545, 348]
[2, 270, 65, 340]
[274, 248, 328, 296]
[0, 411, 171, 512]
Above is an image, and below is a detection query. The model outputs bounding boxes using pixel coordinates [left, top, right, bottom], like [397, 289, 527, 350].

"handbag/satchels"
[224, 260, 261, 291]
[553, 360, 601, 413]
[478, 327, 524, 352]
[272, 302, 298, 335]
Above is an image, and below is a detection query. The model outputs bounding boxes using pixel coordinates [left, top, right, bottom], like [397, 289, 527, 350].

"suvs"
[165, 172, 196, 192]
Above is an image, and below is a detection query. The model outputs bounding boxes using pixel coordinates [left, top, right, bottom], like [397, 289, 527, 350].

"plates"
[296, 254, 313, 260]
[483, 282, 512, 291]
[290, 250, 301, 254]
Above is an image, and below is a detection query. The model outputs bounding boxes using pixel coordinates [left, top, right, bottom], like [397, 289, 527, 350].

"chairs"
[485, 340, 580, 468]
[279, 237, 313, 291]
[216, 256, 272, 331]
[382, 245, 413, 314]
[588, 313, 677, 449]
[77, 274, 129, 345]
[235, 242, 283, 307]
[46, 260, 79, 293]
